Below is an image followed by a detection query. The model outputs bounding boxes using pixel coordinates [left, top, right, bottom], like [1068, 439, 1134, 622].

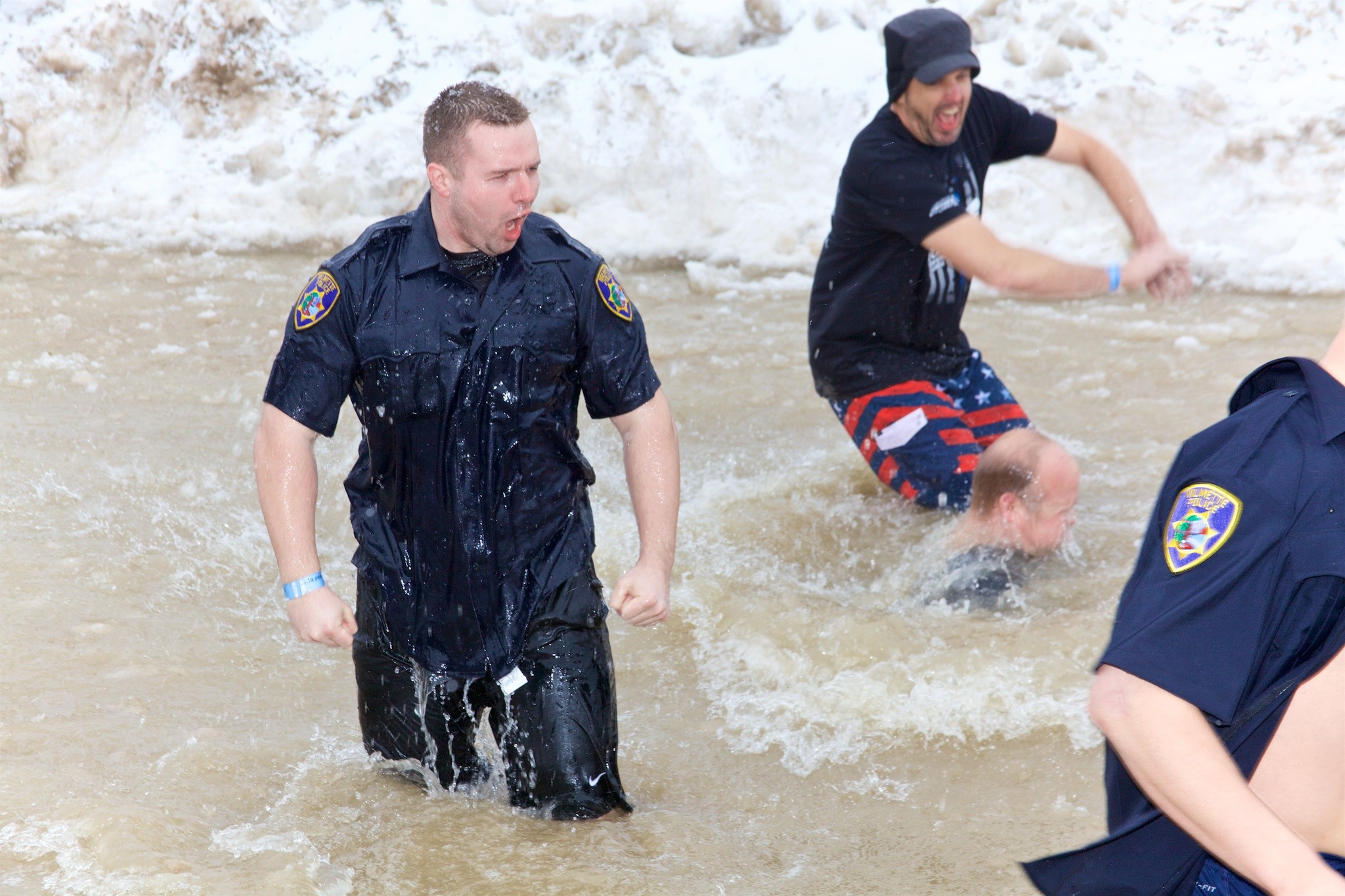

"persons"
[254, 80, 681, 820]
[1016, 311, 1345, 896]
[898, 429, 1078, 611]
[808, 5, 1196, 509]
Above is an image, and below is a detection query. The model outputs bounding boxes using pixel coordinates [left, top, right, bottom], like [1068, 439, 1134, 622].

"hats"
[883, 6, 980, 103]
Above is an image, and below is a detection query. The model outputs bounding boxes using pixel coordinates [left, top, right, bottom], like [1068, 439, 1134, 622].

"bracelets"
[284, 571, 325, 602]
[1099, 259, 1120, 294]
